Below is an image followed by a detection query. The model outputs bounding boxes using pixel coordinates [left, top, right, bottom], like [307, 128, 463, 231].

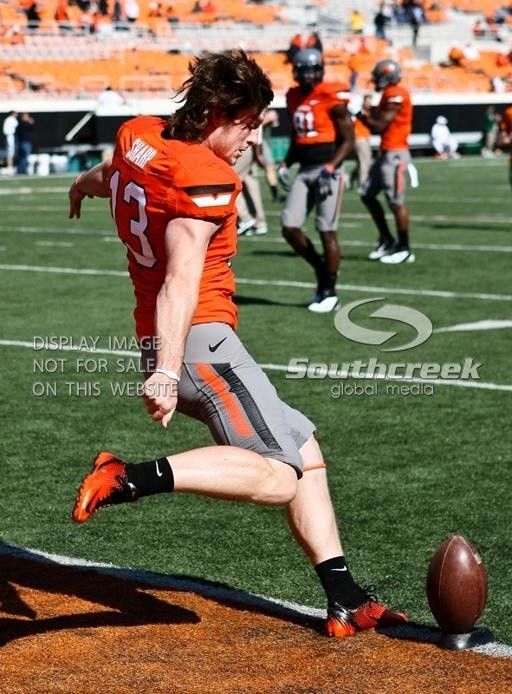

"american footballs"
[426, 537, 489, 634]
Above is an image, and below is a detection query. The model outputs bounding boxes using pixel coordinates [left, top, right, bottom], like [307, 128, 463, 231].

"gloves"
[277, 168, 292, 191]
[313, 171, 333, 203]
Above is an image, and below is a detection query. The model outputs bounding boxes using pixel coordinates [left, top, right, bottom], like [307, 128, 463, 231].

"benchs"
[2, 1, 512, 108]
[370, 140, 486, 160]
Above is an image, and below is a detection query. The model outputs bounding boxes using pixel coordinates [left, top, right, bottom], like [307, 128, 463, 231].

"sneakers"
[380, 249, 416, 265]
[70, 450, 129, 525]
[236, 216, 256, 234]
[369, 235, 399, 260]
[325, 595, 410, 640]
[246, 224, 269, 237]
[308, 295, 341, 315]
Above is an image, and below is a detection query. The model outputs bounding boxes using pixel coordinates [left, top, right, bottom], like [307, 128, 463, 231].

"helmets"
[371, 59, 401, 92]
[293, 48, 325, 85]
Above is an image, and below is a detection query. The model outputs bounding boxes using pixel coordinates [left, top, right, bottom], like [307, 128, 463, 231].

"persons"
[69, 48, 410, 639]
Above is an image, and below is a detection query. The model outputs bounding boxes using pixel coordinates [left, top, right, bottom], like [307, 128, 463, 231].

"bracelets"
[153, 368, 180, 384]
